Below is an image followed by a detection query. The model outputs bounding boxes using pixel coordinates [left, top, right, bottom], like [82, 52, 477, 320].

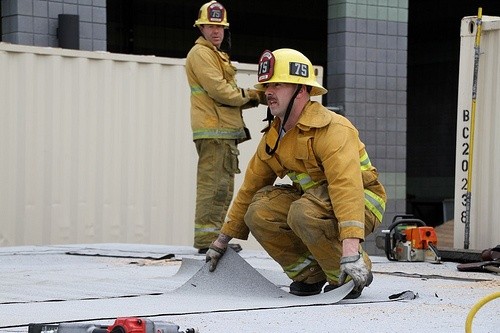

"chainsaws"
[374, 213, 441, 265]
[27, 317, 196, 333]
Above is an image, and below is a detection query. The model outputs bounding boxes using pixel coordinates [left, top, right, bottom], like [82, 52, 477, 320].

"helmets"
[196, 0, 230, 29]
[254, 48, 328, 96]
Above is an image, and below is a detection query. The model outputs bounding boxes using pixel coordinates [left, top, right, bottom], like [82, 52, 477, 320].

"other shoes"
[198, 243, 243, 255]
[290, 276, 327, 297]
[324, 270, 373, 299]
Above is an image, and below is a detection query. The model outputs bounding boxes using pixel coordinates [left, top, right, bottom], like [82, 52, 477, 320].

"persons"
[186, 1, 268, 253]
[204, 49, 387, 299]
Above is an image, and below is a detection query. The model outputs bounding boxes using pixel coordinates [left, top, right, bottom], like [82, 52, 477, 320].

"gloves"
[338, 252, 369, 290]
[205, 239, 229, 272]
